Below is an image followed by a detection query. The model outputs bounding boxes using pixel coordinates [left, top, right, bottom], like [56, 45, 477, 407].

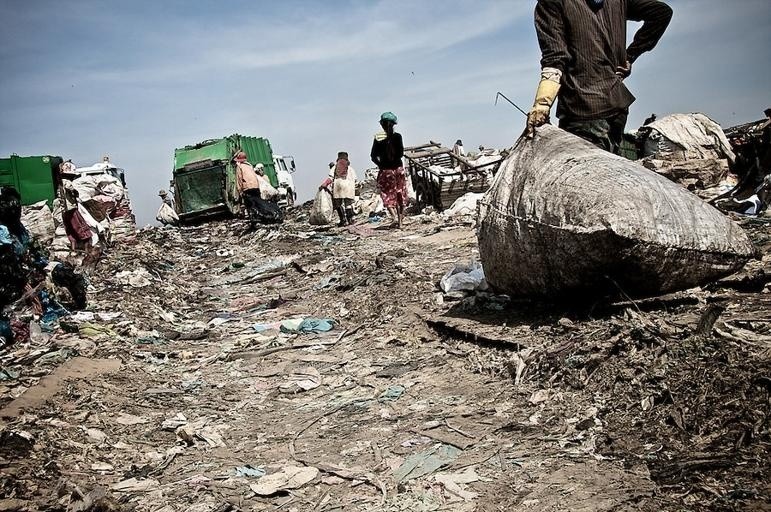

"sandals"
[389, 217, 399, 228]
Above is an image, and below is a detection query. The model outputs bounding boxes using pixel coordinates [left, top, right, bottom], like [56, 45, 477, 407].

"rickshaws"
[394, 134, 491, 215]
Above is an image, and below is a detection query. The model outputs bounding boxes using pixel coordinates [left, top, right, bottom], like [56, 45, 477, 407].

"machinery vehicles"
[0, 150, 129, 216]
[168, 135, 297, 220]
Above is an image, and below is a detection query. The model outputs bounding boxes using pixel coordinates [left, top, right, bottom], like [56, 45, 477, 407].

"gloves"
[528, 79, 562, 139]
[318, 178, 332, 191]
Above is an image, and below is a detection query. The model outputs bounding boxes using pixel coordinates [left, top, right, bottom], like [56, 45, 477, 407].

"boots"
[345, 209, 352, 225]
[336, 206, 346, 226]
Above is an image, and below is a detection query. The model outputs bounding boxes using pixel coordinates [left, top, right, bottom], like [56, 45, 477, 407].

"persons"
[448, 140, 468, 188]
[329, 161, 335, 169]
[526, 1, 674, 156]
[254, 162, 283, 220]
[318, 152, 358, 226]
[158, 189, 172, 208]
[479, 145, 485, 151]
[1, 186, 68, 303]
[234, 152, 277, 233]
[370, 112, 409, 230]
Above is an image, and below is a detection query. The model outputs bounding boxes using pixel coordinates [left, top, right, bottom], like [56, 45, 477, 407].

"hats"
[233, 152, 246, 162]
[381, 112, 397, 124]
[157, 190, 167, 195]
[336, 152, 348, 161]
